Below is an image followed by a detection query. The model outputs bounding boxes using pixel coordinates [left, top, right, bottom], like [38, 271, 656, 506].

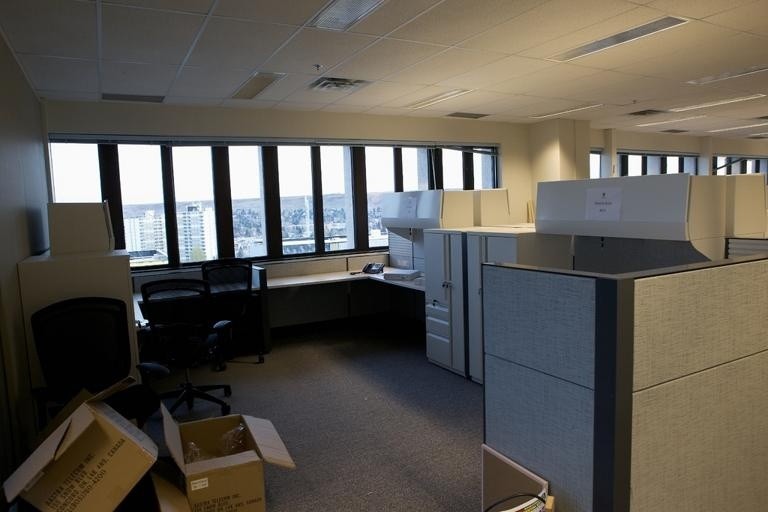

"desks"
[130, 252, 425, 328]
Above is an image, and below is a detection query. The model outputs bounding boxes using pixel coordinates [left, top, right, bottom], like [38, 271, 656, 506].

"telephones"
[363, 262, 385, 274]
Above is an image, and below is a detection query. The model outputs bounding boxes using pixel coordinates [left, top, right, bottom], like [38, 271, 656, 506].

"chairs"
[29, 258, 262, 436]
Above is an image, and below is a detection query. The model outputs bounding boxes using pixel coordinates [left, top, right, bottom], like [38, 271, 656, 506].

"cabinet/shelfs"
[419, 230, 572, 386]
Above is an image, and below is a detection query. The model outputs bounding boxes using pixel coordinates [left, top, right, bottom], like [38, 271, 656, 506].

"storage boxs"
[1, 375, 296, 512]
